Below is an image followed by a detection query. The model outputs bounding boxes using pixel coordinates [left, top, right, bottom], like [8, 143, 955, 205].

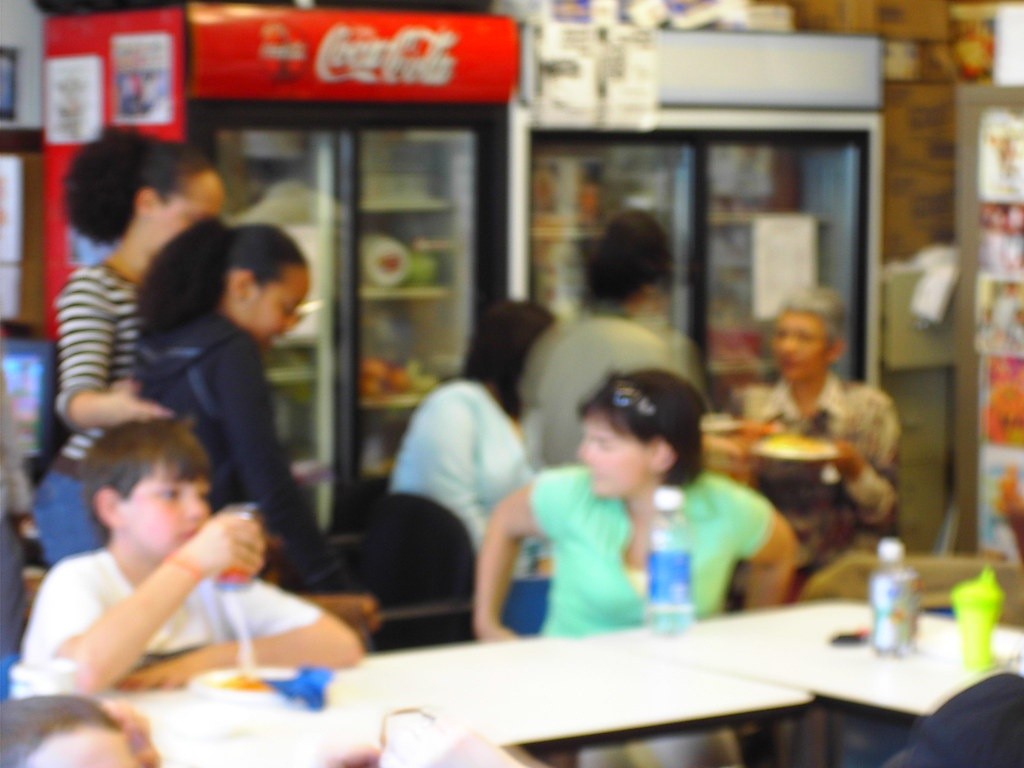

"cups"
[949, 579, 1005, 670]
[216, 510, 259, 592]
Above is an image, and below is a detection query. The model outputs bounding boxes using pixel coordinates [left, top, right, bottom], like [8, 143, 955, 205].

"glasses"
[608, 379, 659, 422]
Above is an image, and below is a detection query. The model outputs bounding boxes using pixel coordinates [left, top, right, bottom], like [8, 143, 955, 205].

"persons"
[18, 419, 365, 696]
[32, 127, 225, 569]
[725, 290, 900, 600]
[515, 206, 710, 469]
[129, 219, 354, 599]
[388, 299, 549, 582]
[471, 367, 794, 768]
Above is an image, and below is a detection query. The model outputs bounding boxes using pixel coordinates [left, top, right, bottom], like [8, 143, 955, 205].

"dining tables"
[329, 643, 817, 766]
[671, 595, 1023, 766]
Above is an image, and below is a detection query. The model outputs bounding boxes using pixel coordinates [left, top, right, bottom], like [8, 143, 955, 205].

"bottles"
[357, 233, 409, 285]
[868, 538, 918, 651]
[648, 488, 693, 634]
[533, 159, 601, 220]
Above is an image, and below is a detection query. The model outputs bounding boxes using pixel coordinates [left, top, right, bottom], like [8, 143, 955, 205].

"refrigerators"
[36, 0, 882, 613]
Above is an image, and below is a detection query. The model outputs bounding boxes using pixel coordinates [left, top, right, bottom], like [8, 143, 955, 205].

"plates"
[747, 433, 836, 460]
